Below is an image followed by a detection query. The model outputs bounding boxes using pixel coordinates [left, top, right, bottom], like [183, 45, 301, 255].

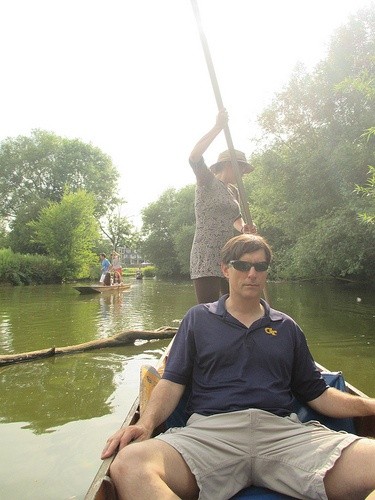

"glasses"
[230, 260, 269, 272]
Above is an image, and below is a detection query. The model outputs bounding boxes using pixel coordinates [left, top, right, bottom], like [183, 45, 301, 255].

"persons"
[189, 108, 257, 304]
[110, 250, 122, 286]
[100, 233, 375, 500]
[100, 253, 112, 287]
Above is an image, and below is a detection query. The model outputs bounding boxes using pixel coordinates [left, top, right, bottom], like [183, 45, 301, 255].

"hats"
[210, 149, 255, 175]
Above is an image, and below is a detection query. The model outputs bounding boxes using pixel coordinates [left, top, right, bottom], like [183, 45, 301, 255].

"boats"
[72, 281, 131, 294]
[135, 272, 143, 279]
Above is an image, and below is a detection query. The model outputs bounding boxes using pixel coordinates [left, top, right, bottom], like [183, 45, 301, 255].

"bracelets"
[240, 227, 244, 233]
